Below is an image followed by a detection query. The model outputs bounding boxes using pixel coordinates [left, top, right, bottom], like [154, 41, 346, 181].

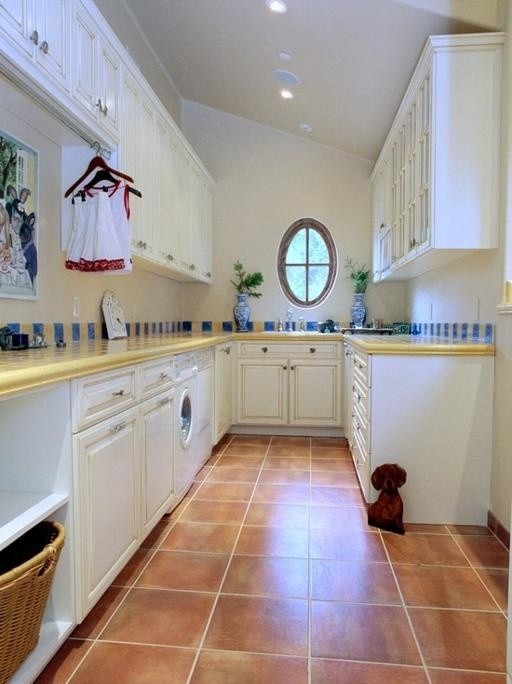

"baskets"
[0, 518, 65, 684]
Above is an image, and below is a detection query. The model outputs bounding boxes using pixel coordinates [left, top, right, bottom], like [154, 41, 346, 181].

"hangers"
[62, 141, 143, 199]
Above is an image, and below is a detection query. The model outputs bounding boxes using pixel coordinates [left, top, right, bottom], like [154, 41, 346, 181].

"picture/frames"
[0, 127, 38, 302]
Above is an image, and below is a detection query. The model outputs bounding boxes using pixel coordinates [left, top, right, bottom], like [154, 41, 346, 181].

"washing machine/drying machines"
[166, 352, 194, 515]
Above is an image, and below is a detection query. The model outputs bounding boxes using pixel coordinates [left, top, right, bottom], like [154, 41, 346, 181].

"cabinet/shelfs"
[158, 108, 183, 269]
[373, 39, 501, 283]
[68, 355, 174, 618]
[0, 0, 72, 102]
[183, 136, 204, 280]
[72, 0, 119, 134]
[203, 165, 215, 286]
[343, 343, 496, 527]
[236, 339, 344, 429]
[215, 341, 235, 450]
[3, 377, 76, 682]
[120, 57, 158, 262]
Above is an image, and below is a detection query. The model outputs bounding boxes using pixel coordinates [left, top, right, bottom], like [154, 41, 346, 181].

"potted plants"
[344, 257, 373, 327]
[230, 261, 265, 333]
